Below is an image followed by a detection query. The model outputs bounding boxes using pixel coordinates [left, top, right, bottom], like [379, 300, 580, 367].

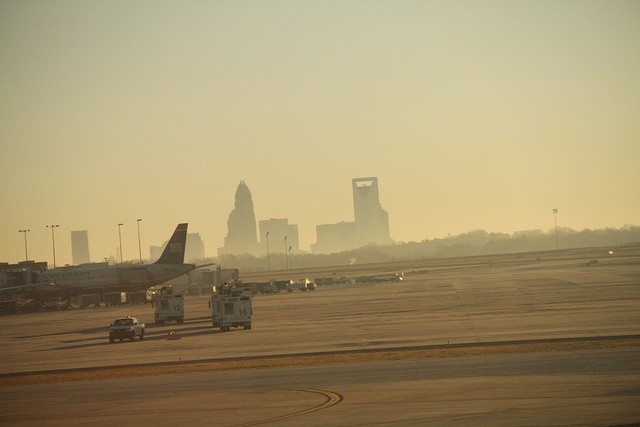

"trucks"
[220, 294, 252, 331]
[208, 295, 221, 326]
[152, 295, 184, 323]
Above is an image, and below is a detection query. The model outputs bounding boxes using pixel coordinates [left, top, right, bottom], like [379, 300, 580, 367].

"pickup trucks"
[109, 316, 145, 340]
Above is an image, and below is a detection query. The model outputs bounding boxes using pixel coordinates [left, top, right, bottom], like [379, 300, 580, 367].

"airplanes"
[35, 223, 216, 303]
